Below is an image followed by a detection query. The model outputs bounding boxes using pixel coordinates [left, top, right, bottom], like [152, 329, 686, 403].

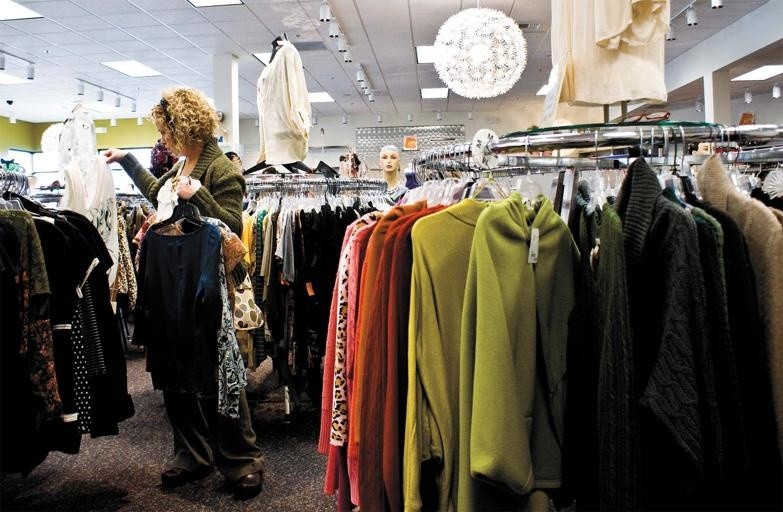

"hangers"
[149, 194, 202, 233]
[241, 176, 391, 213]
[0, 168, 31, 212]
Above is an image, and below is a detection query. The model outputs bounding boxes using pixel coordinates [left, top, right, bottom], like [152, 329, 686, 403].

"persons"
[377, 144, 410, 202]
[99, 85, 265, 497]
[690, 140, 714, 157]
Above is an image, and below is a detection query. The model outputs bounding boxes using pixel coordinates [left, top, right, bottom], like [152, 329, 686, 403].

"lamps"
[431, 0, 530, 101]
[320, 0, 375, 103]
[667, 0, 723, 42]
[692, 82, 782, 112]
[0, 49, 143, 127]
[311, 111, 473, 126]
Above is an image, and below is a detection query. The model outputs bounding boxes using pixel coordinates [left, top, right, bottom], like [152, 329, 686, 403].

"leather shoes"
[234, 471, 264, 499]
[161, 462, 214, 488]
